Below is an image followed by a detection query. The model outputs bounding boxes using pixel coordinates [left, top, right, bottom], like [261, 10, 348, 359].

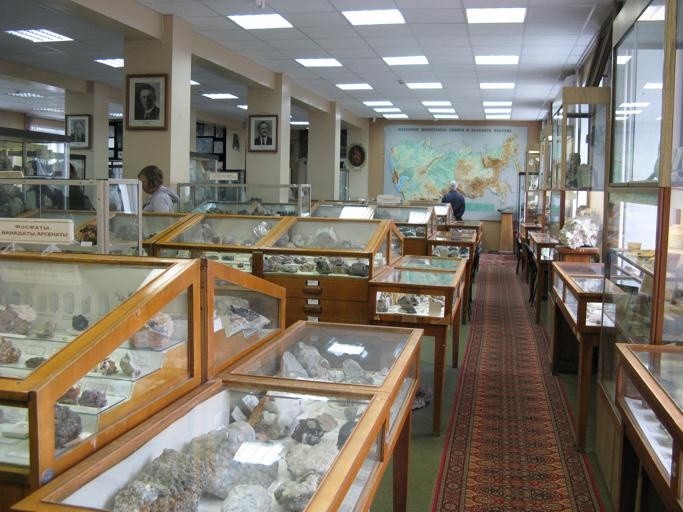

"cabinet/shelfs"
[518, 2, 682, 512]
[2, 167, 481, 512]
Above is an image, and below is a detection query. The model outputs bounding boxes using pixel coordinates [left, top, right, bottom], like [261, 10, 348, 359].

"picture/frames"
[63, 112, 91, 150]
[247, 114, 277, 153]
[347, 143, 367, 167]
[125, 72, 167, 132]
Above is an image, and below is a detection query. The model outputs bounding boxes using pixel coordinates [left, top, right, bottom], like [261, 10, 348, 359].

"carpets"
[429, 248, 606, 511]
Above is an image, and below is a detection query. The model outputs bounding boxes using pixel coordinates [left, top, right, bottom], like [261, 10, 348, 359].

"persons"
[71, 119, 84, 142]
[350, 147, 363, 166]
[254, 120, 272, 145]
[135, 84, 159, 120]
[441, 180, 465, 220]
[138, 165, 179, 256]
